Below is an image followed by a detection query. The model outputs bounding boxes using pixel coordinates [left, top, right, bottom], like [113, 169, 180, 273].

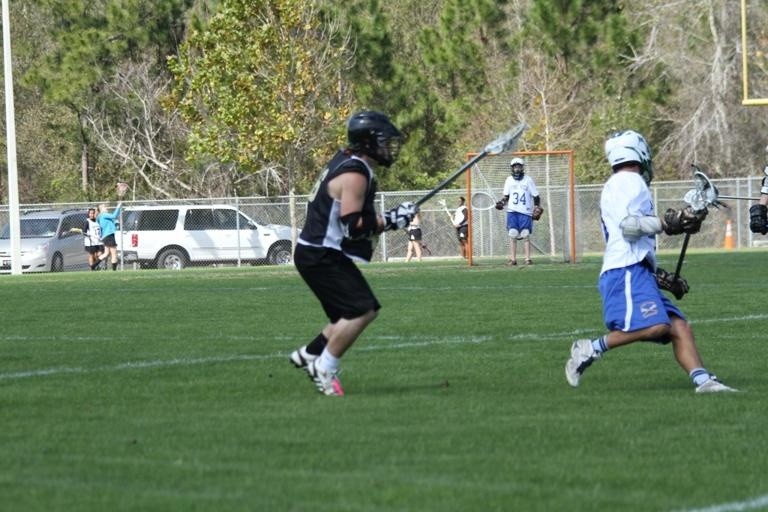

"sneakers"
[505, 259, 532, 264]
[694, 375, 739, 393]
[289, 345, 345, 396]
[565, 339, 603, 387]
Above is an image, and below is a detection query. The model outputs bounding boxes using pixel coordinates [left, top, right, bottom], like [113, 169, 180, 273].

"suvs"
[109, 203, 303, 270]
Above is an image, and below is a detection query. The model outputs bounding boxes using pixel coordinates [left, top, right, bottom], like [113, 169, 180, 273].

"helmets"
[604, 129, 651, 169]
[348, 111, 403, 167]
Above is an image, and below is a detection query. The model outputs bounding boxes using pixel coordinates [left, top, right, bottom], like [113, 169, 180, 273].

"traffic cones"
[721, 220, 737, 250]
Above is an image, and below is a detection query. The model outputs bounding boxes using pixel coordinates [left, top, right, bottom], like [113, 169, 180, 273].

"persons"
[453, 196, 470, 258]
[564, 127, 739, 396]
[91, 199, 124, 271]
[494, 157, 542, 266]
[405, 208, 422, 263]
[83, 207, 104, 271]
[285, 110, 419, 398]
[749, 159, 768, 238]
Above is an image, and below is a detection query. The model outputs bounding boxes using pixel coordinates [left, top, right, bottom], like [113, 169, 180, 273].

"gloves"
[664, 203, 708, 235]
[749, 204, 768, 235]
[381, 201, 419, 232]
[655, 267, 689, 301]
[495, 198, 505, 210]
[532, 206, 543, 220]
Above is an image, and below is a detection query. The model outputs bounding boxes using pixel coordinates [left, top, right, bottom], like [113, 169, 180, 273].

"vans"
[0, 208, 99, 275]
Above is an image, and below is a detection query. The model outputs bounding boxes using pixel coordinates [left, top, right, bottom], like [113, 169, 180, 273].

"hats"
[511, 157, 523, 167]
